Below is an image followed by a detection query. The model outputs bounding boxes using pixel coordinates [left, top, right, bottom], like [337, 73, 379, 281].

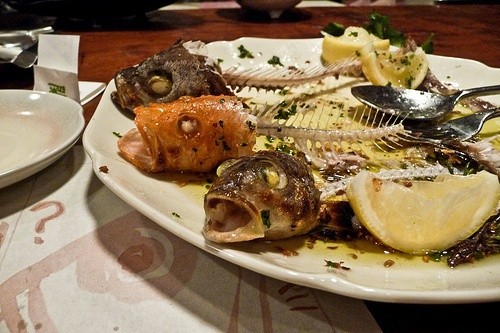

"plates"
[81, 37, 500, 304]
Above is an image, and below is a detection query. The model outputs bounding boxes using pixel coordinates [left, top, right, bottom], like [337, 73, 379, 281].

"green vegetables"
[323, 13, 433, 54]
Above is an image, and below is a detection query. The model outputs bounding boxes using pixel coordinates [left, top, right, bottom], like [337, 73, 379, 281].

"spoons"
[350, 85, 499, 120]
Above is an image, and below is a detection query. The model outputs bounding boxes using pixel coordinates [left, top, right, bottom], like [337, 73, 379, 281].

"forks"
[382, 106, 499, 149]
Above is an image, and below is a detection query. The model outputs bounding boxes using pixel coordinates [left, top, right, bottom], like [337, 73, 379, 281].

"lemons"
[346, 170, 499, 255]
[322, 26, 391, 72]
[360, 44, 428, 90]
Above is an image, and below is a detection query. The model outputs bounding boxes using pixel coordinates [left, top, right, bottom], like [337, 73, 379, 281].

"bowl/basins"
[236, 0, 301, 18]
[0, 88, 85, 189]
